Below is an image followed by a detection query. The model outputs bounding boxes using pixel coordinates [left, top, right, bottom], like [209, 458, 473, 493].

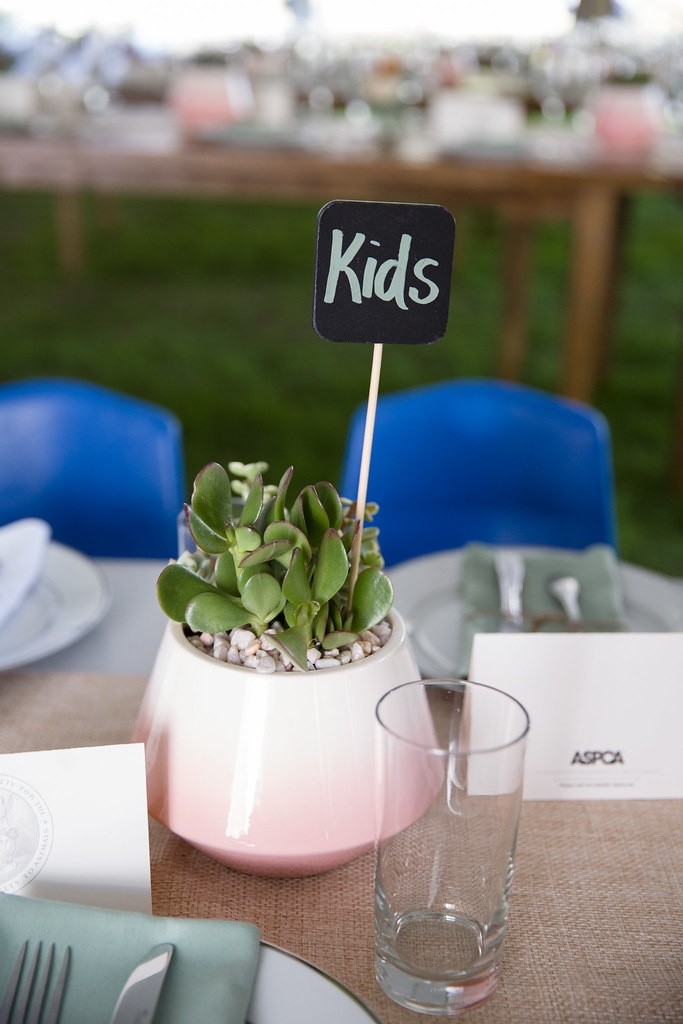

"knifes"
[108, 943, 177, 1024]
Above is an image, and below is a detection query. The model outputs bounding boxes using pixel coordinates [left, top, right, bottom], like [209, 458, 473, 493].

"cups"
[374, 678, 523, 1016]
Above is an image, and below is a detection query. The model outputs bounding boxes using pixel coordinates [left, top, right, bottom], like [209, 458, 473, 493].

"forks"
[1, 941, 75, 1024]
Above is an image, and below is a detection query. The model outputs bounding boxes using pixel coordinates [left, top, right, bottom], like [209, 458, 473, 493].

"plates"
[382, 546, 682, 700]
[242, 939, 391, 1024]
[0, 521, 110, 674]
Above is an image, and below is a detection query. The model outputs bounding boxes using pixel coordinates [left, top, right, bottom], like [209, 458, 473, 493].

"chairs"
[340, 380, 620, 571]
[0, 378, 187, 561]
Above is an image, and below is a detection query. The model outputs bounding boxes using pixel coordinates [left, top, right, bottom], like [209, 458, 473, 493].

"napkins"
[453, 543, 629, 680]
[0, 891, 260, 1024]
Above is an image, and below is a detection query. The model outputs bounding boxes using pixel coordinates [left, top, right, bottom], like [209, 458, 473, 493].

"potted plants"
[135, 460, 445, 881]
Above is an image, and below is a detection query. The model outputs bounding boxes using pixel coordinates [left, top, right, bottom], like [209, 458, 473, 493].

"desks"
[0, 562, 683, 1024]
